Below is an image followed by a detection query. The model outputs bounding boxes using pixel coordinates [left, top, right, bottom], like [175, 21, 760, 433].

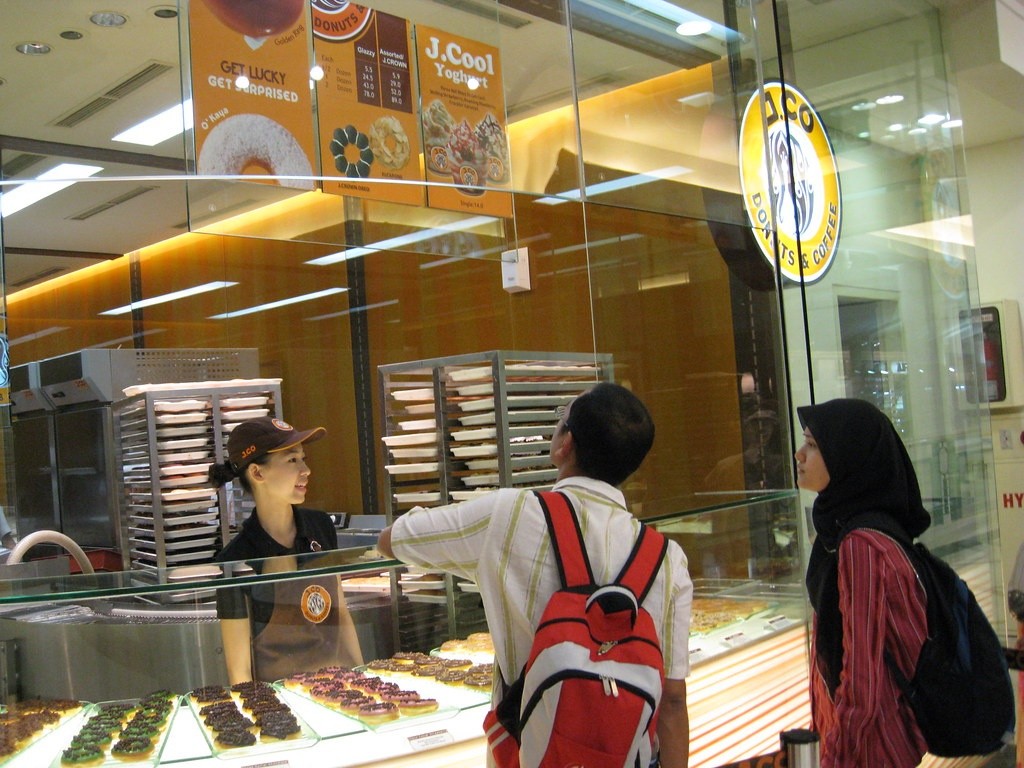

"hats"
[227, 416, 327, 474]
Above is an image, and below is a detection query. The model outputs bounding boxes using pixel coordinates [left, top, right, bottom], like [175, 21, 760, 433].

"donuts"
[196, 111, 315, 190]
[284, 664, 438, 725]
[364, 631, 496, 694]
[200, 0, 307, 37]
[689, 599, 767, 635]
[189, 680, 302, 749]
[329, 125, 374, 178]
[0, 688, 179, 768]
[369, 116, 411, 171]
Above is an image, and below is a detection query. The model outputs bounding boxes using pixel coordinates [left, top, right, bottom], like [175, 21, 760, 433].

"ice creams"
[421, 99, 511, 196]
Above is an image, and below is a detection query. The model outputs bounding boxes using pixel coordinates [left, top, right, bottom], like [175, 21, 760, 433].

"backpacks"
[482, 489, 670, 768]
[829, 520, 1018, 757]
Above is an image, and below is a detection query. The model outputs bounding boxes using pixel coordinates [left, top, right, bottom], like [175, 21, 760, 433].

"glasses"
[555, 405, 574, 443]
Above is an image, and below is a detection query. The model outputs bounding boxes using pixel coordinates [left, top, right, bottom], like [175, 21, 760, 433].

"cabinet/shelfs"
[0, 488, 817, 768]
[374, 350, 618, 654]
[111, 376, 282, 609]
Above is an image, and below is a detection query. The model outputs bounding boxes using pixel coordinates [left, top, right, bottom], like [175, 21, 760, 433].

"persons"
[377, 382, 693, 768]
[795, 398, 930, 768]
[0, 507, 16, 548]
[208, 417, 364, 686]
[1009, 542, 1024, 653]
